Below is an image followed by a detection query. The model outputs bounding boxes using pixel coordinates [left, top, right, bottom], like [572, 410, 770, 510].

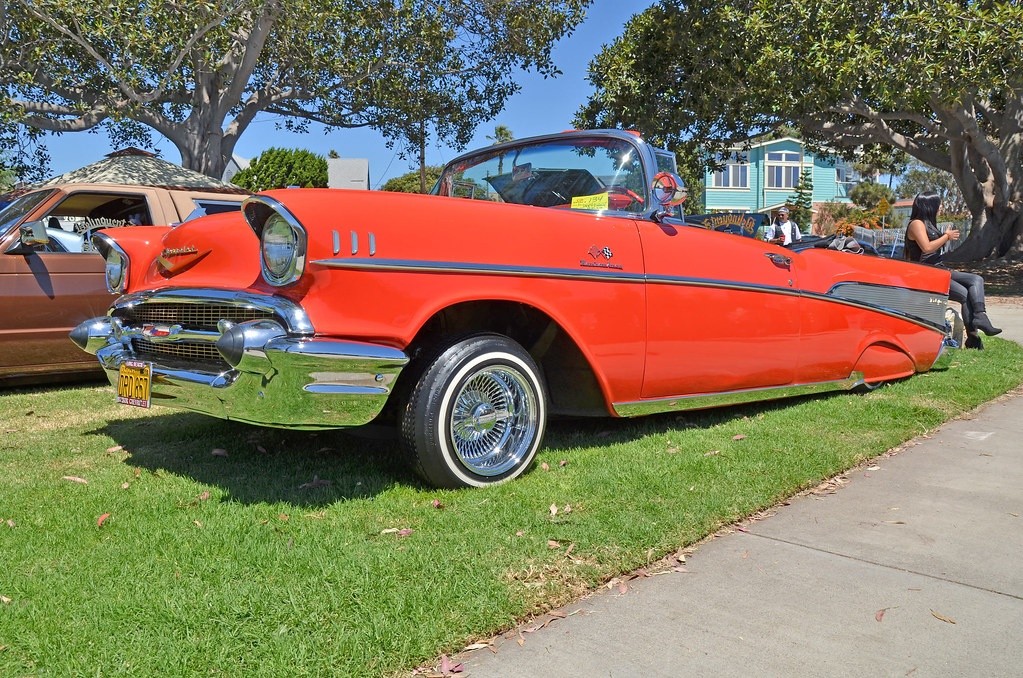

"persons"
[905, 191, 1002, 350]
[764, 207, 802, 245]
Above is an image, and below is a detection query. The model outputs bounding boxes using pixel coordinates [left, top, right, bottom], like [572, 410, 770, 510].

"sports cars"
[66, 127, 963, 492]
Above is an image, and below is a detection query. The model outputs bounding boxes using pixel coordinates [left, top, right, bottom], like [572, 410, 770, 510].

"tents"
[0, 146, 254, 213]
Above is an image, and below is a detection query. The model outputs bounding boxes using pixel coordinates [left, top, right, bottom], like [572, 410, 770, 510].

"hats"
[778, 207, 789, 213]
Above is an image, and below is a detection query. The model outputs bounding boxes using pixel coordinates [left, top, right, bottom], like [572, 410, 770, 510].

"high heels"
[972, 312, 1002, 336]
[965, 334, 984, 351]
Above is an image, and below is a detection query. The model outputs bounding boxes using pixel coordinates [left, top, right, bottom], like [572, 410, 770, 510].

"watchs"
[944, 233, 951, 241]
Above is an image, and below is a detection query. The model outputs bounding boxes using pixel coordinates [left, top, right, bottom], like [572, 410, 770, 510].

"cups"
[780, 235, 785, 242]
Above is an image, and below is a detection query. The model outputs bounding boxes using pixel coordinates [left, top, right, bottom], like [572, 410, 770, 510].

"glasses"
[778, 214, 784, 216]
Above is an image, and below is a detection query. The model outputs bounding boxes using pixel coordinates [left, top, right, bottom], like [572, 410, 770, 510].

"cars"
[0, 178, 255, 385]
[482, 166, 907, 261]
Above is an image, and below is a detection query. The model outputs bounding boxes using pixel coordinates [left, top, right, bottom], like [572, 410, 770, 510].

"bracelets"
[767, 238, 769, 243]
